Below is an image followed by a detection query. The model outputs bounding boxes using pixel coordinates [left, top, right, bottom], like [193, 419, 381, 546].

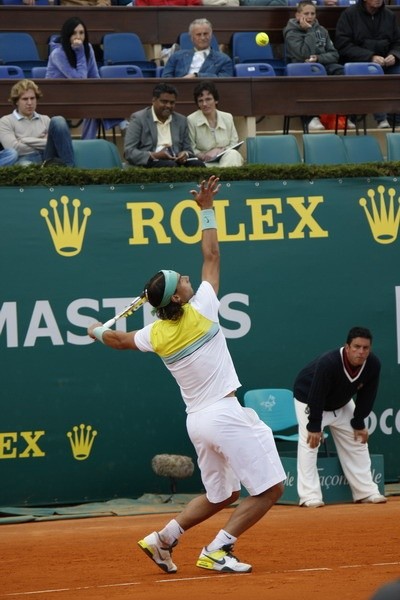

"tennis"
[256, 31, 268, 48]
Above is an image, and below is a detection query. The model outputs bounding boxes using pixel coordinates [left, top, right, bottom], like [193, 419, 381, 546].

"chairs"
[99, 65, 144, 146]
[342, 135, 384, 164]
[103, 33, 156, 78]
[343, 63, 397, 136]
[179, 31, 219, 50]
[0, 33, 43, 78]
[32, 67, 46, 78]
[243, 388, 330, 457]
[303, 134, 348, 164]
[234, 63, 286, 134]
[0, 66, 24, 78]
[286, 62, 339, 134]
[72, 139, 121, 170]
[246, 135, 302, 165]
[232, 31, 281, 73]
[386, 132, 400, 162]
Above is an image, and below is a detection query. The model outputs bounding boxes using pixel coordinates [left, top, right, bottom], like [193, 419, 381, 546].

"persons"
[281, 0, 340, 64]
[85, 174, 287, 576]
[45, 16, 130, 139]
[291, 327, 389, 510]
[185, 81, 244, 168]
[0, 80, 77, 166]
[123, 83, 206, 168]
[331, 1, 400, 66]
[162, 16, 232, 77]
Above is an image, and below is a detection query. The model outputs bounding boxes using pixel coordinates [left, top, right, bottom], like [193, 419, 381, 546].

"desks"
[0, 77, 253, 139]
[0, 4, 158, 44]
[388, 3, 399, 17]
[160, 6, 349, 44]
[253, 73, 400, 135]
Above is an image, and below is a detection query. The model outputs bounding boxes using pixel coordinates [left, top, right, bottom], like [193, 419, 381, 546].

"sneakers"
[358, 493, 388, 503]
[300, 500, 325, 508]
[137, 531, 179, 573]
[306, 119, 326, 130]
[196, 542, 252, 573]
